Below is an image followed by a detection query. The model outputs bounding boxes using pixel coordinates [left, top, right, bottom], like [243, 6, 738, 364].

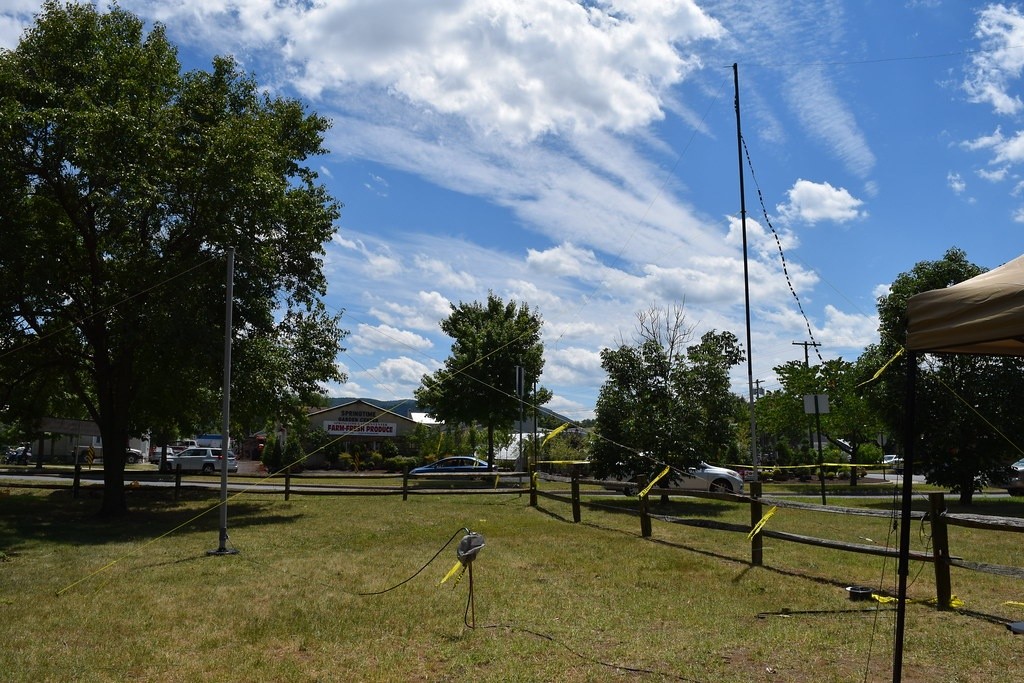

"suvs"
[159, 447, 239, 473]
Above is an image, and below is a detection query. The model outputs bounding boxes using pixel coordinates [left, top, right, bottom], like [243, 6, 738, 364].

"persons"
[5, 442, 32, 466]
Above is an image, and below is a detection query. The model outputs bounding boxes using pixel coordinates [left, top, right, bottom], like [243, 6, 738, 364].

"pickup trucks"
[69, 443, 143, 465]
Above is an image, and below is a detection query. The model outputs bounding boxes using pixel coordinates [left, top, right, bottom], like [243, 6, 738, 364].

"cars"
[601, 451, 744, 494]
[880, 453, 905, 468]
[409, 456, 499, 475]
[152, 447, 175, 465]
[1003, 458, 1024, 497]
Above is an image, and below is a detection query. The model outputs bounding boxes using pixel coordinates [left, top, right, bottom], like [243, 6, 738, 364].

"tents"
[894, 251, 1024, 682]
